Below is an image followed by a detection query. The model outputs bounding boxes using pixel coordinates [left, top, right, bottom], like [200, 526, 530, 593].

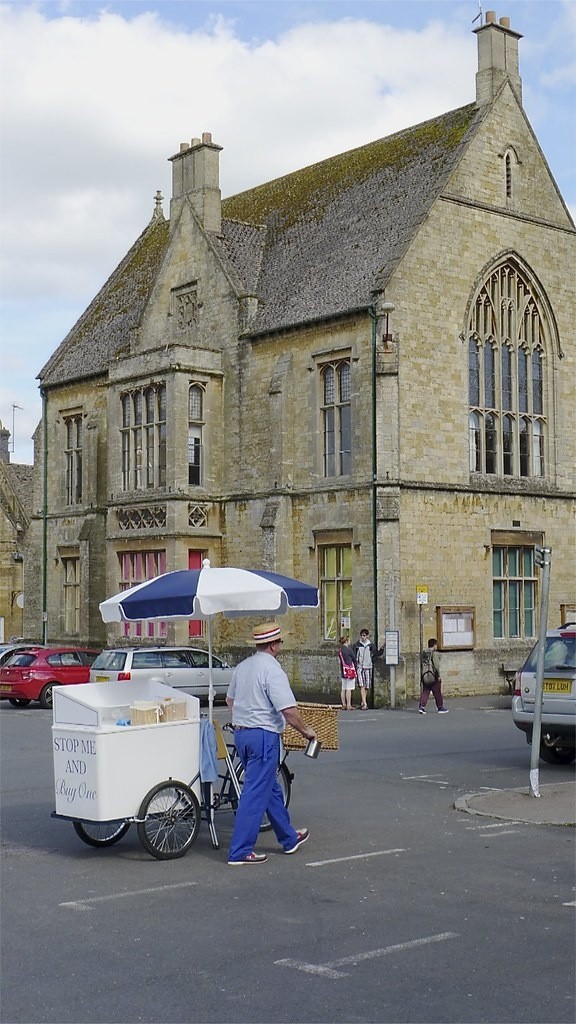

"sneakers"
[419, 706, 426, 715]
[438, 706, 449, 714]
[285, 827, 310, 854]
[227, 848, 267, 865]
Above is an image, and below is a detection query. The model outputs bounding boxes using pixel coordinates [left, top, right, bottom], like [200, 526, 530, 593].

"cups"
[304, 739, 322, 759]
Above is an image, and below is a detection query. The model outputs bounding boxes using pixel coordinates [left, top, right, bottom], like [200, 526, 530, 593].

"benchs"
[501, 663, 518, 694]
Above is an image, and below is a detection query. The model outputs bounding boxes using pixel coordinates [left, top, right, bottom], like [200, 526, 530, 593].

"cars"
[0, 643, 78, 665]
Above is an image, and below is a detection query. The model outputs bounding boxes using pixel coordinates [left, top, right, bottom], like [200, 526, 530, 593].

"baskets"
[280, 701, 339, 751]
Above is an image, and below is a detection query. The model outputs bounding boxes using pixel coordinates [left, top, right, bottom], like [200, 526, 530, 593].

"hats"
[245, 622, 282, 644]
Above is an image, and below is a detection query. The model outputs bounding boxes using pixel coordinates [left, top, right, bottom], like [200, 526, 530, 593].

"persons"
[226, 622, 316, 865]
[419, 639, 450, 714]
[338, 637, 357, 710]
[353, 629, 385, 710]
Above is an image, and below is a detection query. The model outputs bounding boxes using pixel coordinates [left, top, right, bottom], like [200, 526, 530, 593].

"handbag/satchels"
[342, 664, 357, 679]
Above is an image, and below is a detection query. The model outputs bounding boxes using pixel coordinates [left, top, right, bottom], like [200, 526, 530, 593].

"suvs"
[0, 647, 121, 710]
[89, 644, 236, 702]
[509, 620, 576, 765]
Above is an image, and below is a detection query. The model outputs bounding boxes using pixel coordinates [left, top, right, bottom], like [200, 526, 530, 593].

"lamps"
[381, 303, 395, 342]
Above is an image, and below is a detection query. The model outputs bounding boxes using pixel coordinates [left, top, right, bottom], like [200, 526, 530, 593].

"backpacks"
[420, 651, 435, 686]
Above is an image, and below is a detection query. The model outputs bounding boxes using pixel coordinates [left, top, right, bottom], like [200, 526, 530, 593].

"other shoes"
[342, 704, 355, 710]
[361, 703, 368, 710]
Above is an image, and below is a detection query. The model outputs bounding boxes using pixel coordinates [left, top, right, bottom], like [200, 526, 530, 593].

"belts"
[234, 726, 250, 730]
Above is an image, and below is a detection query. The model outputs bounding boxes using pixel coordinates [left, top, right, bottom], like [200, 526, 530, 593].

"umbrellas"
[98, 558, 319, 725]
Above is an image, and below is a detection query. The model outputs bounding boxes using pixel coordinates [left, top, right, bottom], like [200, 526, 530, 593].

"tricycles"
[51, 721, 295, 862]
